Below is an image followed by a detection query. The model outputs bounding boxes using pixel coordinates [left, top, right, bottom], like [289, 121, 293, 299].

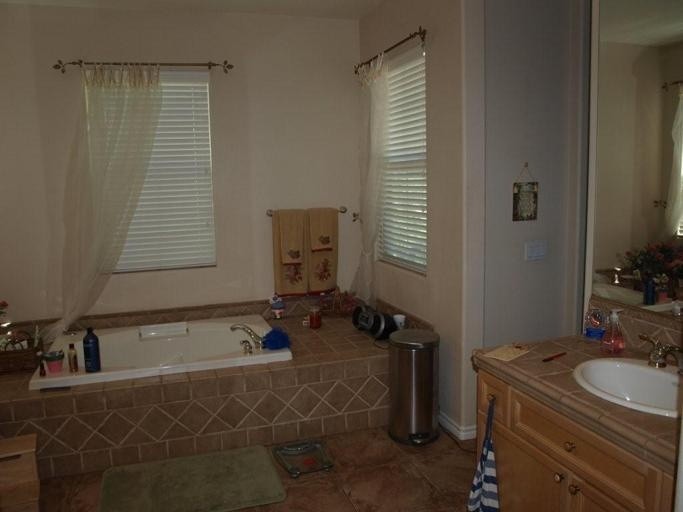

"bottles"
[39, 362, 46, 376]
[308, 305, 323, 330]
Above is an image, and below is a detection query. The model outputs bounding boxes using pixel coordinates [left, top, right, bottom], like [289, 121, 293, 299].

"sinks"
[574, 357, 682, 419]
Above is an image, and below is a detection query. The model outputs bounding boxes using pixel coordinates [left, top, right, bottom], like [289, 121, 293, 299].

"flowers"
[0, 300, 8, 316]
[616, 230, 682, 312]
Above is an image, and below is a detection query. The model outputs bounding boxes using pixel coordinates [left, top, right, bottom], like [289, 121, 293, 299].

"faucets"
[658, 343, 682, 371]
[231, 324, 262, 348]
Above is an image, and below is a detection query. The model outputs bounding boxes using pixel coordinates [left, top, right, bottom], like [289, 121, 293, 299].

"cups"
[43, 350, 65, 374]
[393, 314, 405, 330]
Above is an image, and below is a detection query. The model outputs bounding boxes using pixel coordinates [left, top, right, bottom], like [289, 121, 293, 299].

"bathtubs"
[29, 312, 294, 392]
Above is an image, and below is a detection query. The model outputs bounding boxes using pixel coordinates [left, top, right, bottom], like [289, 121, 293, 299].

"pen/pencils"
[543, 353, 566, 362]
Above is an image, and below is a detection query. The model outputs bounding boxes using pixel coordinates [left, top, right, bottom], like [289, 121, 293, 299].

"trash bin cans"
[388, 329, 440, 445]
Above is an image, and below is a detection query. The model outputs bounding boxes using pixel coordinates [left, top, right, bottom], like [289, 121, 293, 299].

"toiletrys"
[83, 326, 101, 373]
[601, 308, 627, 354]
[39, 364, 46, 375]
[311, 309, 319, 328]
[68, 342, 78, 373]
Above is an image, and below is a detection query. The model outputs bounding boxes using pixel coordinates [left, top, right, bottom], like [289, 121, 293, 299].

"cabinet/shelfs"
[471, 358, 677, 512]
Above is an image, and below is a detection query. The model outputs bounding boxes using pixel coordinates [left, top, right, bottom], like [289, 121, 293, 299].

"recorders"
[352, 305, 399, 341]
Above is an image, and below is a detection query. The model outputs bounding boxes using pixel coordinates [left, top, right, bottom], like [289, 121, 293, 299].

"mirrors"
[580, 0, 681, 321]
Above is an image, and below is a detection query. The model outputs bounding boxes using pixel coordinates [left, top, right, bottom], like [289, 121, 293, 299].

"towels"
[306, 206, 339, 297]
[272, 209, 311, 299]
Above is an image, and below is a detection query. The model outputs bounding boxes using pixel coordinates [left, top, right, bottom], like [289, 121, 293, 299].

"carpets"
[98, 444, 286, 512]
